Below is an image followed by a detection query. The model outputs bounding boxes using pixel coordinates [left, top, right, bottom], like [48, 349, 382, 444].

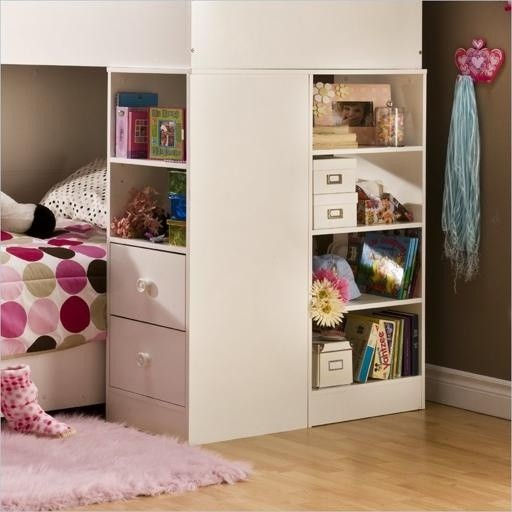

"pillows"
[38, 154, 106, 228]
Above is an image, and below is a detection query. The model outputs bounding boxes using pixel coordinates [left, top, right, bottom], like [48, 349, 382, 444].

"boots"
[0, 365, 76, 438]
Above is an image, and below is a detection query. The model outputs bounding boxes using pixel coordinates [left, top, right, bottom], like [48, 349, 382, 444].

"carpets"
[0, 409, 253, 512]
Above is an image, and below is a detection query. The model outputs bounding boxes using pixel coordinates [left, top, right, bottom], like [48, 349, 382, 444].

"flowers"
[309, 270, 349, 328]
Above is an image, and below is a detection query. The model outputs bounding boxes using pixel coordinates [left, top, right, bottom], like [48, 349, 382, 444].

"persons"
[336, 103, 370, 126]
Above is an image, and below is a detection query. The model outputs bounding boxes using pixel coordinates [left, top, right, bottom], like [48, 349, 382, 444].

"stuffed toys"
[0, 191, 57, 238]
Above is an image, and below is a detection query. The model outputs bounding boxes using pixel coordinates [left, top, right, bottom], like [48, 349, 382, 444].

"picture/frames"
[312, 83, 393, 145]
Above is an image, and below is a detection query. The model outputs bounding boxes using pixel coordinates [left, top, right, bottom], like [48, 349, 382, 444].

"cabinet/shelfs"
[106, 68, 426, 446]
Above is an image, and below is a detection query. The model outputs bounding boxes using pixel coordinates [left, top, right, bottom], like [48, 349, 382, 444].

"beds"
[0, 203, 106, 420]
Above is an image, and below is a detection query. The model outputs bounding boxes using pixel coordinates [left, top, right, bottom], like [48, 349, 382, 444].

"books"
[313, 126, 358, 150]
[344, 232, 421, 383]
